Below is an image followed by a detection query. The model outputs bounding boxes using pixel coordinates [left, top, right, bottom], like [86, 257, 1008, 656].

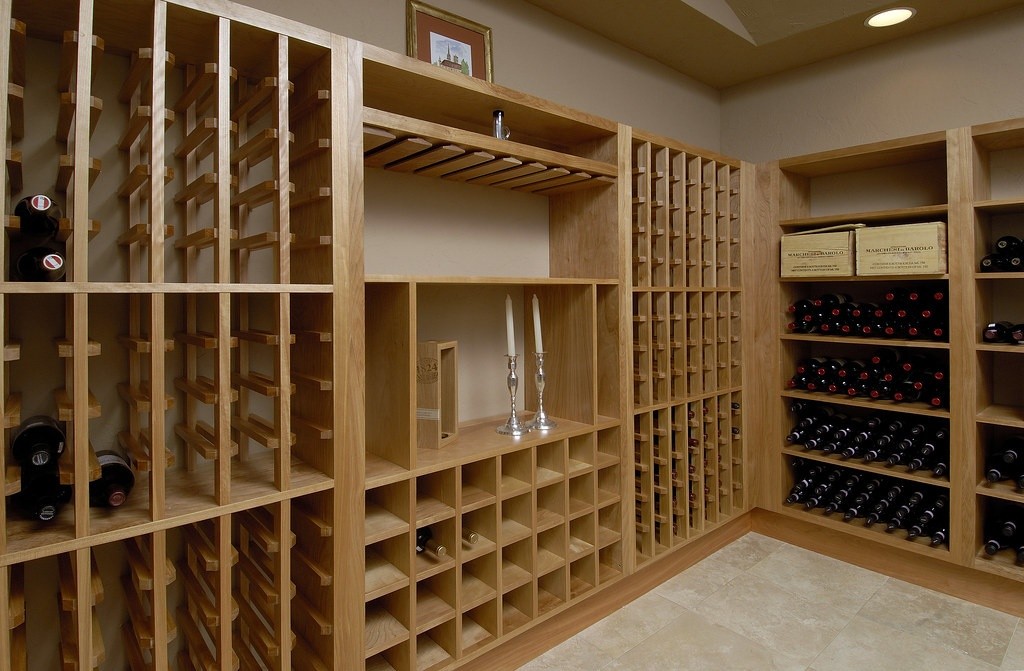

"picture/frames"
[407, 0, 493, 85]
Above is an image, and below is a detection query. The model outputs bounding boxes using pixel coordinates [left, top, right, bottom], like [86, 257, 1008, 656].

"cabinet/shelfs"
[0, 0, 758, 670]
[761, 119, 1024, 619]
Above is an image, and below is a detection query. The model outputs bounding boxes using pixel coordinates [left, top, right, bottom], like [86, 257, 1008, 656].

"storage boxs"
[416, 340, 460, 448]
[856, 220, 948, 279]
[780, 222, 864, 280]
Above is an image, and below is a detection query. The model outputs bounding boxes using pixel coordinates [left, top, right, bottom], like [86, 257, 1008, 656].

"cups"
[492, 109, 510, 140]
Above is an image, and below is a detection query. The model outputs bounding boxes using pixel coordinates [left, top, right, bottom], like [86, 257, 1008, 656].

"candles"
[505, 295, 516, 356]
[532, 294, 544, 353]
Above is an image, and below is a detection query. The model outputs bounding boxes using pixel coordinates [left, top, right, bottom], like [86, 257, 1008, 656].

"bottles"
[783, 455, 950, 546]
[783, 286, 948, 341]
[462, 528, 478, 544]
[982, 321, 1024, 343]
[980, 235, 1024, 273]
[982, 513, 1024, 567]
[983, 439, 1024, 493]
[416, 527, 447, 558]
[788, 351, 949, 408]
[786, 401, 949, 480]
[13, 416, 135, 529]
[15, 194, 67, 282]
[654, 402, 740, 533]
